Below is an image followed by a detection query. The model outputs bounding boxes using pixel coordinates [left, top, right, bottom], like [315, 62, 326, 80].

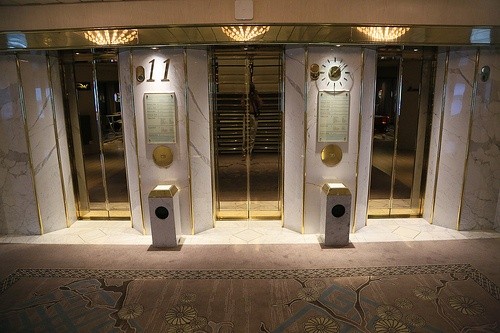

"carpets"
[0, 264, 500, 333]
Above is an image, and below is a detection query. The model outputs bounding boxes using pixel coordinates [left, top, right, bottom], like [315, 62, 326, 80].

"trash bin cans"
[147, 184, 182, 248]
[320, 183, 353, 246]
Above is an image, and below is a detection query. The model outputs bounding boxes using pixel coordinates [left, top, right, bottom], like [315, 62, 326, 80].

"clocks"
[320, 55, 352, 90]
